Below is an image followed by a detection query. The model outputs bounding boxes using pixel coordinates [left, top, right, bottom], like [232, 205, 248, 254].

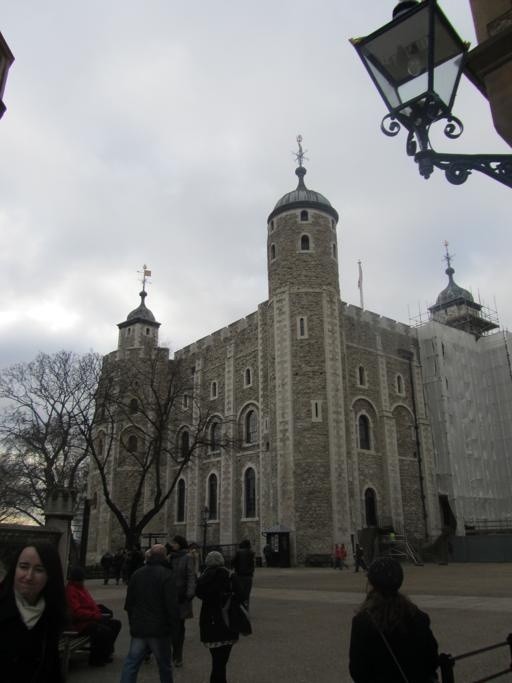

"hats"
[145, 543, 169, 568]
[205, 551, 225, 564]
[174, 535, 188, 548]
[369, 558, 403, 593]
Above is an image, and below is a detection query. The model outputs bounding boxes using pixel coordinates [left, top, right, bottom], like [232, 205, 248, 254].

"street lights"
[200, 506, 213, 564]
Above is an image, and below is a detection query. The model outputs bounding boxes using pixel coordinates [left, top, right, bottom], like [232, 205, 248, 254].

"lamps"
[348, 0, 511, 188]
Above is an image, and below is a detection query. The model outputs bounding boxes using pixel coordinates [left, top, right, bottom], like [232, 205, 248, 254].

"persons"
[64, 565, 123, 667]
[340, 542, 350, 567]
[263, 541, 275, 567]
[348, 556, 440, 682]
[101, 534, 258, 682]
[354, 544, 369, 572]
[0, 537, 67, 682]
[334, 542, 343, 569]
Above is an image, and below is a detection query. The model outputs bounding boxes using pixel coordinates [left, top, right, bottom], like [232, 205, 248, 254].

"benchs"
[58, 631, 92, 682]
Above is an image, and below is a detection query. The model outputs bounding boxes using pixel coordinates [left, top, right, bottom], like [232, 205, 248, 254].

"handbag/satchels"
[222, 595, 252, 636]
